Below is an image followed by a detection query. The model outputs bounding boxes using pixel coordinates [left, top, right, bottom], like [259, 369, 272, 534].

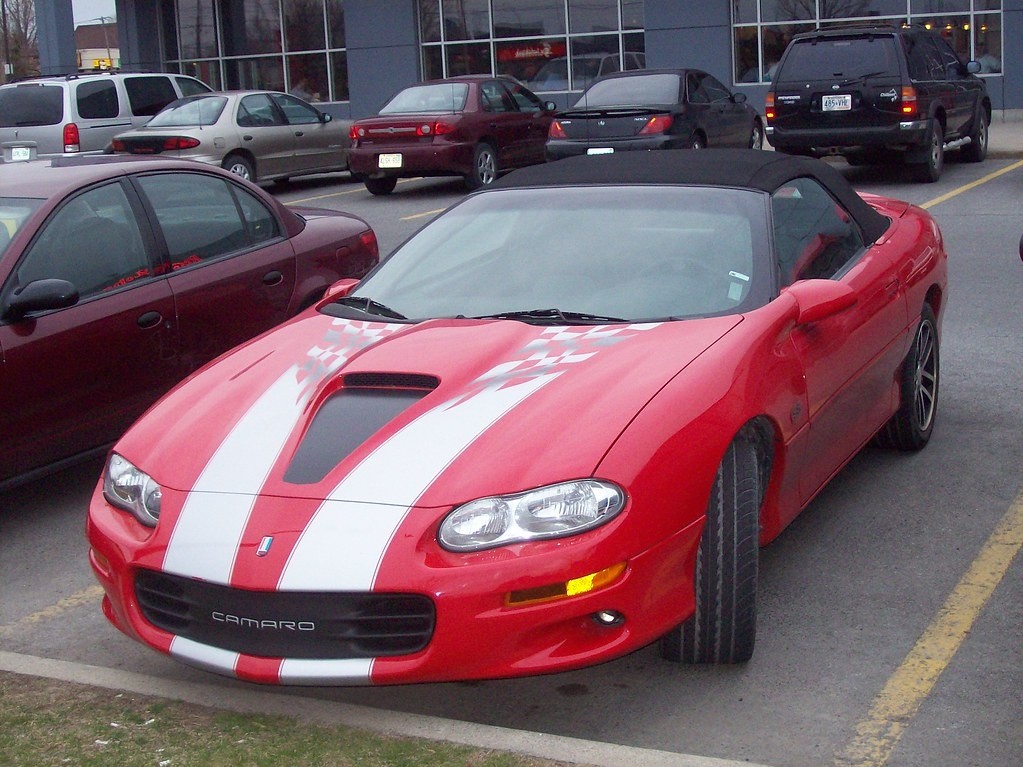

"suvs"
[763, 23, 993, 183]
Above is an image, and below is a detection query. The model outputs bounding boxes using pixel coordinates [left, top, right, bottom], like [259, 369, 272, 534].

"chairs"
[56, 215, 130, 296]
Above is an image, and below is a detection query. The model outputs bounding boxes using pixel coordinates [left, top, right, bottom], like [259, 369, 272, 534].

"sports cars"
[85, 146, 950, 688]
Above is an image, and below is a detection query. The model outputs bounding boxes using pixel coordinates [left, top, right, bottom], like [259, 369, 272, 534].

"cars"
[348, 78, 562, 196]
[0, 152, 380, 493]
[527, 49, 646, 92]
[544, 68, 764, 164]
[110, 91, 367, 187]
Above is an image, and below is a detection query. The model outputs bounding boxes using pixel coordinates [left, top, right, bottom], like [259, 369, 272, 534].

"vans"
[0, 67, 217, 164]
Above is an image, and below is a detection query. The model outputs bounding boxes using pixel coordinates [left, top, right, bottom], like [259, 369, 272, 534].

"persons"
[973, 43, 1001, 74]
[291, 77, 316, 102]
[743, 60, 781, 82]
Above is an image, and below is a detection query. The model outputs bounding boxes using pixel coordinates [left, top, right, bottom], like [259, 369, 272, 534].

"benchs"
[126, 222, 243, 268]
